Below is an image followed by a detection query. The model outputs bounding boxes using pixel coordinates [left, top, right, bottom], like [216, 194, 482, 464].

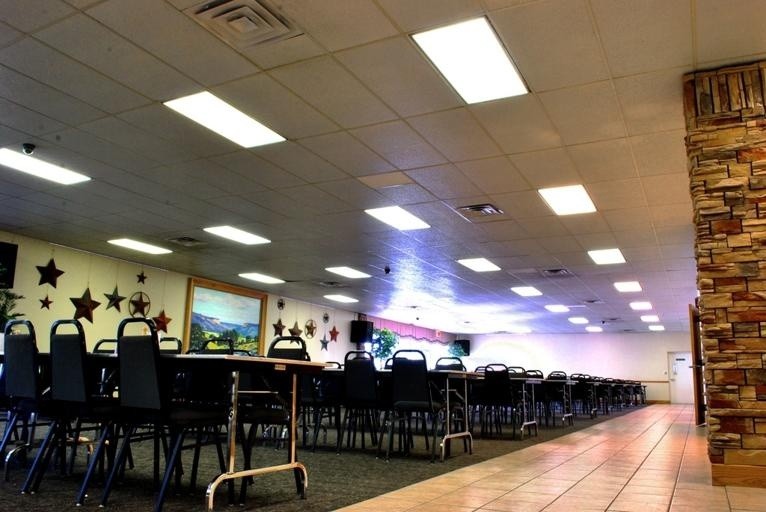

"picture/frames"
[181, 274, 269, 357]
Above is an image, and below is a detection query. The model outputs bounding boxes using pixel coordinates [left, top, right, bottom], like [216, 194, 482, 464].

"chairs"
[470, 364, 576, 439]
[571, 373, 646, 418]
[341, 350, 484, 463]
[0, 318, 341, 511]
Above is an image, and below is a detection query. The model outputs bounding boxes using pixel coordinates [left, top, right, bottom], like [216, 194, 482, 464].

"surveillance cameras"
[21, 143, 35, 155]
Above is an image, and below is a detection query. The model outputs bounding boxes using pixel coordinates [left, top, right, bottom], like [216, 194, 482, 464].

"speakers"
[351, 321, 373, 343]
[454, 340, 470, 356]
[0, 241, 19, 290]
